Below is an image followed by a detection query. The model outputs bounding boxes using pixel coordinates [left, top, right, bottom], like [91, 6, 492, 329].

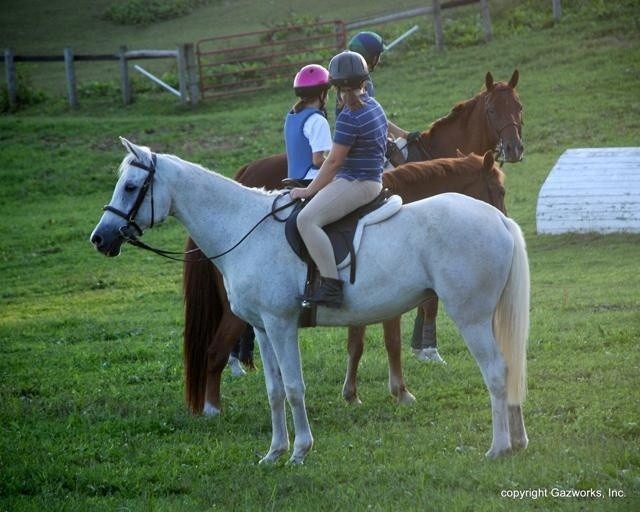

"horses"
[89, 136, 531, 469]
[227, 68, 527, 379]
[181, 147, 509, 420]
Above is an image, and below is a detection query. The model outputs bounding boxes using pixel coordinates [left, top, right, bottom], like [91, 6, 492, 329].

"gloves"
[407, 131, 423, 144]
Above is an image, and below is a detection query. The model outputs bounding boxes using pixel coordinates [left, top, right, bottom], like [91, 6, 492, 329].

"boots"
[294, 277, 346, 310]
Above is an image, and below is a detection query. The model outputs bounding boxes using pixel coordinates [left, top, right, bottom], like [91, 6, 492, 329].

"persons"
[283, 63, 333, 182]
[290, 51, 387, 307]
[335, 32, 421, 171]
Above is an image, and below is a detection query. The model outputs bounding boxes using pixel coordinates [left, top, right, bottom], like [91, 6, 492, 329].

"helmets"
[348, 30, 391, 59]
[326, 50, 371, 93]
[292, 64, 331, 99]
